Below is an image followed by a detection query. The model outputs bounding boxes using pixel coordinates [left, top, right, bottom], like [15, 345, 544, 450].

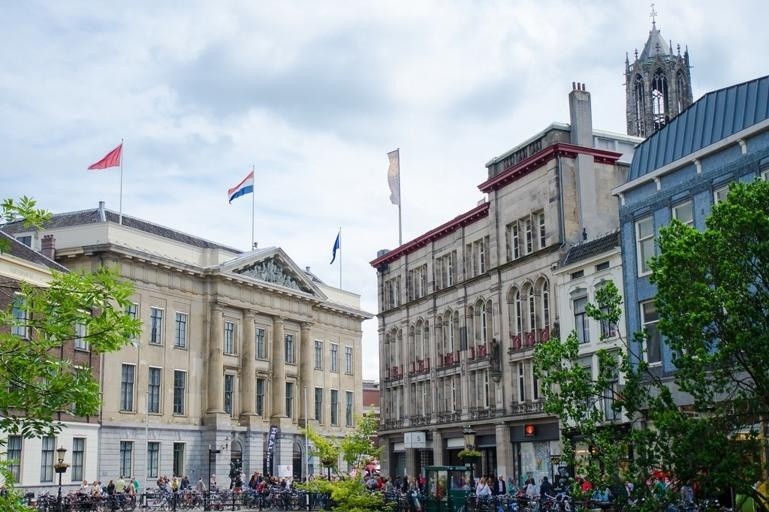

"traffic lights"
[523, 423, 537, 438]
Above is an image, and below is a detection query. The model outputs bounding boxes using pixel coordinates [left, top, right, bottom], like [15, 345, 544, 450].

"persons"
[79, 475, 139, 511]
[157, 473, 229, 511]
[623, 465, 768, 511]
[227, 466, 351, 511]
[453, 471, 615, 511]
[351, 460, 448, 511]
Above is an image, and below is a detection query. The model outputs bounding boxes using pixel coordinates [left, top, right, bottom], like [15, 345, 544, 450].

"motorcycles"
[463, 483, 580, 512]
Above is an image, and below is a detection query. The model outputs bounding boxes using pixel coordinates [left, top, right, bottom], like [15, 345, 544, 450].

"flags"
[330, 231, 341, 265]
[228, 169, 255, 206]
[87, 140, 124, 171]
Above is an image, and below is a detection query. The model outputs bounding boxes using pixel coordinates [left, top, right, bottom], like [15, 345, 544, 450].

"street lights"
[206, 436, 233, 499]
[462, 424, 479, 510]
[52, 444, 68, 512]
